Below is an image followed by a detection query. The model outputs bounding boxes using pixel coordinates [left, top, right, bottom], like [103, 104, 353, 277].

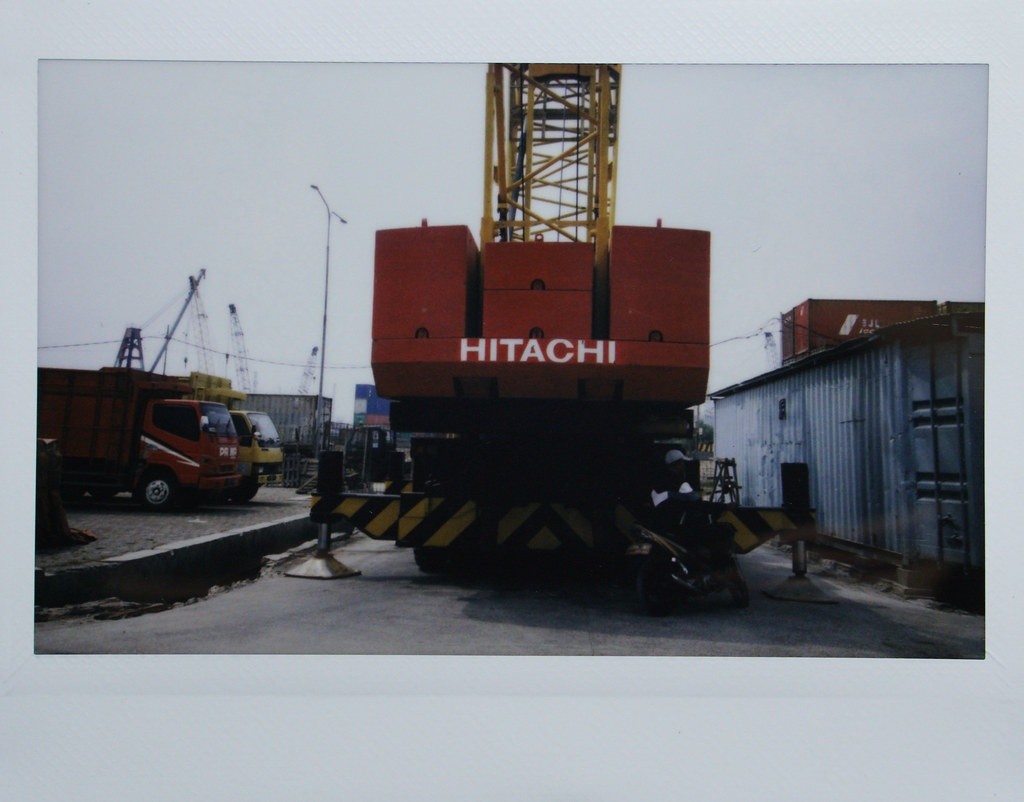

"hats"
[665, 450, 693, 466]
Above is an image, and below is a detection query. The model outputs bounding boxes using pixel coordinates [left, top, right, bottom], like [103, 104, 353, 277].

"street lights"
[310, 184, 348, 458]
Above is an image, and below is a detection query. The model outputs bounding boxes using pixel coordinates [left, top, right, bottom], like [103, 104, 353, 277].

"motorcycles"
[625, 482, 751, 616]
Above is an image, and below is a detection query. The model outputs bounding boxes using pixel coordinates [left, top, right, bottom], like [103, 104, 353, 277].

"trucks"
[37, 366, 244, 512]
[172, 372, 285, 503]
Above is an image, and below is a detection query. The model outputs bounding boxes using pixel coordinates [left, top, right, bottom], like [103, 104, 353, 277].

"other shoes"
[695, 562, 713, 574]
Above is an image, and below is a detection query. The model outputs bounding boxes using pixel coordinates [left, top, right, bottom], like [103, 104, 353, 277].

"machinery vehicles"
[309, 62, 817, 590]
